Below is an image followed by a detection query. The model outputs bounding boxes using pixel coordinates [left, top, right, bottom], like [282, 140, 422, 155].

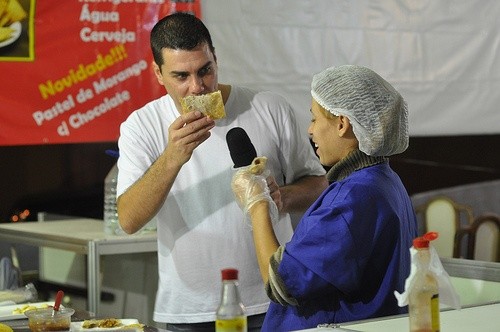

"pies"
[250, 157, 267, 173]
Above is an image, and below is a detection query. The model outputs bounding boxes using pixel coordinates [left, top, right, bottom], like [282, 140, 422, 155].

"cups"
[24, 306, 75, 332]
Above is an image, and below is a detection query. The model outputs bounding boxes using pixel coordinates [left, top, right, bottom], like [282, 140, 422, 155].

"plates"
[70, 319, 140, 332]
[0, 301, 64, 319]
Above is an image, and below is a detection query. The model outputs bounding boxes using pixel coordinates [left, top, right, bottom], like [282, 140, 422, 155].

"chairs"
[415, 197, 500, 264]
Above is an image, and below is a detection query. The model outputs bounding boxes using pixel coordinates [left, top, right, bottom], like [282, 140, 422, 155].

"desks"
[0, 218, 157, 316]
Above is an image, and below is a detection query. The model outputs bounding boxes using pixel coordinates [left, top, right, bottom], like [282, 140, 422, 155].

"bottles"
[215, 269, 248, 332]
[407, 231, 441, 332]
[103, 148, 146, 238]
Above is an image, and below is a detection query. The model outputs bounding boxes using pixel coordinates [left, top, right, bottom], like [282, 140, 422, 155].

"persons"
[116, 12, 329, 332]
[232, 65, 419, 332]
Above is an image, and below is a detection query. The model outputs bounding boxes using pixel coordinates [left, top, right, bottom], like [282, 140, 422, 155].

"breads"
[180, 91, 226, 124]
